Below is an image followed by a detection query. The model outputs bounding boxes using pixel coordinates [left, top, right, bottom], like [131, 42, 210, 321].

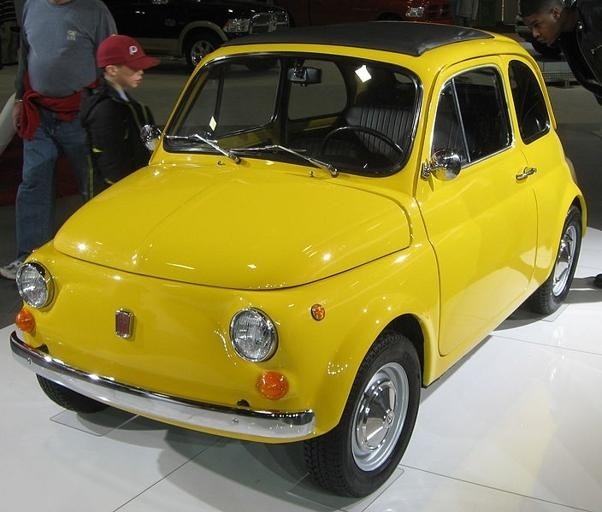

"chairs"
[324, 83, 494, 175]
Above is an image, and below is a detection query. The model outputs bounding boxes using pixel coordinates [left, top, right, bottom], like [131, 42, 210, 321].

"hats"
[95, 34, 160, 69]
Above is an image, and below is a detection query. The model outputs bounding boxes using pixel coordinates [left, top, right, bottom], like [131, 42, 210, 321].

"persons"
[80, 34, 160, 201]
[520, 0, 602, 287]
[1, 1, 117, 279]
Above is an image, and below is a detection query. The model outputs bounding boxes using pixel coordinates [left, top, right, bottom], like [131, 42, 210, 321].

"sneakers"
[0, 259, 23, 280]
[593, 274, 602, 289]
